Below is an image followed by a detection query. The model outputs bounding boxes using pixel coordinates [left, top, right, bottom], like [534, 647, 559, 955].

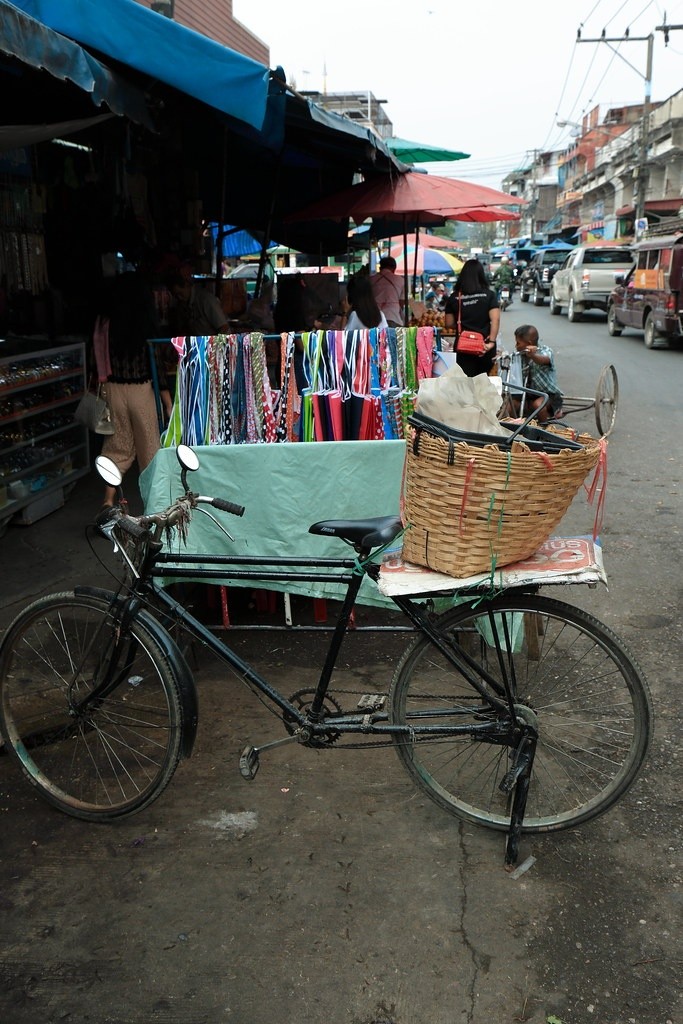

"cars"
[226, 262, 259, 304]
[452, 249, 537, 284]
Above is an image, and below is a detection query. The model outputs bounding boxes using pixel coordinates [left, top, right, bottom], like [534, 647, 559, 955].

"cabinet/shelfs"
[0, 336, 91, 518]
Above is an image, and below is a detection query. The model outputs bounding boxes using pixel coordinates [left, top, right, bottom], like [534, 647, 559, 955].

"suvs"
[519, 247, 574, 306]
[607, 233, 683, 348]
[548, 244, 638, 322]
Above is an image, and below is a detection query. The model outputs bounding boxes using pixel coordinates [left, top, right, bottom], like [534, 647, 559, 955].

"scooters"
[492, 276, 517, 313]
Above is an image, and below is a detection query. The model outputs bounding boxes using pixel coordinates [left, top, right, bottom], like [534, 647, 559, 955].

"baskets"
[401, 425, 604, 578]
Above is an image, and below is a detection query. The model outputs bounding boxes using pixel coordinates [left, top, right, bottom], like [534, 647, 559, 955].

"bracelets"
[489, 340, 496, 343]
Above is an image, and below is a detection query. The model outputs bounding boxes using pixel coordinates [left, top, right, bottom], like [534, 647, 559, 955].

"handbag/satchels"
[456, 331, 485, 355]
[75, 391, 115, 436]
[496, 354, 524, 395]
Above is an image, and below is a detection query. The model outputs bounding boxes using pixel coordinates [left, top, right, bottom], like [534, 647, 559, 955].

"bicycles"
[1, 444, 654, 873]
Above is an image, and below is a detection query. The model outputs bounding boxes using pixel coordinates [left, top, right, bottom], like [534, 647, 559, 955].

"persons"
[473, 254, 560, 302]
[445, 259, 500, 376]
[492, 325, 564, 430]
[85, 270, 175, 523]
[151, 257, 450, 371]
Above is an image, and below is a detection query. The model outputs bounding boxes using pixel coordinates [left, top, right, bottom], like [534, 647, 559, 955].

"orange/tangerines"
[408, 310, 455, 334]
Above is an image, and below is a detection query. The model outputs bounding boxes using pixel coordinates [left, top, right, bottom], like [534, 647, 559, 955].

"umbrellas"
[486, 237, 576, 265]
[266, 137, 530, 328]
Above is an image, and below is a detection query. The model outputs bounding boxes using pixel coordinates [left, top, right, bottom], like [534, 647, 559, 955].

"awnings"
[0, 0, 428, 175]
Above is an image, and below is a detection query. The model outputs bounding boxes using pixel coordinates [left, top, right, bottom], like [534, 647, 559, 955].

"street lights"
[558, 116, 649, 243]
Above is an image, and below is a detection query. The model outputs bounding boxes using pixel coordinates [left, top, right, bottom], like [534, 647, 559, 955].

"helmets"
[501, 257, 510, 265]
